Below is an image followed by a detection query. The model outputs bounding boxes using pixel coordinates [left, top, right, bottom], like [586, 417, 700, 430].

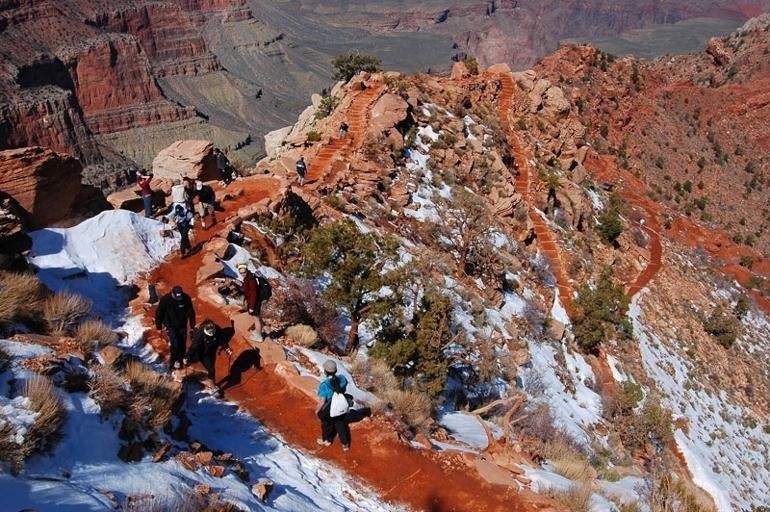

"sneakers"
[206, 379, 215, 389]
[174, 358, 188, 369]
[179, 248, 192, 259]
[343, 445, 349, 451]
[250, 330, 263, 343]
[317, 439, 331, 447]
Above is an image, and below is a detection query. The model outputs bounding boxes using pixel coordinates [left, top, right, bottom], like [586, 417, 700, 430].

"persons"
[165, 170, 216, 259]
[296, 157, 308, 187]
[181, 317, 232, 380]
[155, 285, 197, 369]
[136, 168, 155, 219]
[235, 264, 264, 343]
[315, 360, 351, 451]
[339, 121, 348, 139]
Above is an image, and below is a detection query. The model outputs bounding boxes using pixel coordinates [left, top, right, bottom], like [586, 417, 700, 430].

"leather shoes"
[249, 273, 272, 302]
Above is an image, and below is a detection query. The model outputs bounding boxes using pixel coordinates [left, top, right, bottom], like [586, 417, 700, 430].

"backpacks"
[223, 157, 230, 168]
[329, 392, 349, 417]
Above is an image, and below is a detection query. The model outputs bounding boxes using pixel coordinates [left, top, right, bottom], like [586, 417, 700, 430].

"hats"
[235, 262, 248, 270]
[197, 181, 202, 191]
[172, 286, 183, 300]
[322, 361, 337, 373]
